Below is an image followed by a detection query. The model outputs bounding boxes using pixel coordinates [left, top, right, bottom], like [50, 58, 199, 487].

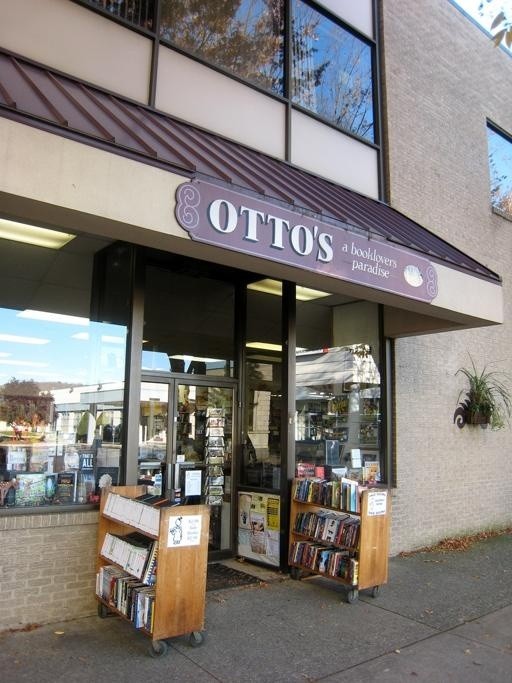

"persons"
[251, 514, 265, 535]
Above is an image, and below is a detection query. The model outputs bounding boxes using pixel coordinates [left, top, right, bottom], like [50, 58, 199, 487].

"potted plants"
[453, 351, 511, 432]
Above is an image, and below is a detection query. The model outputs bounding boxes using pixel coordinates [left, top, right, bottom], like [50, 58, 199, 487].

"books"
[290, 474, 378, 589]
[95, 489, 176, 634]
[3, 441, 124, 504]
[180, 401, 231, 542]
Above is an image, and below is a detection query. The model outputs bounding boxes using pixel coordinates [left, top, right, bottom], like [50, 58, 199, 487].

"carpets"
[205, 562, 266, 595]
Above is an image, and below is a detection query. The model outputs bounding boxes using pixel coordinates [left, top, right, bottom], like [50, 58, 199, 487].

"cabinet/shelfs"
[287, 477, 391, 604]
[93, 483, 211, 657]
